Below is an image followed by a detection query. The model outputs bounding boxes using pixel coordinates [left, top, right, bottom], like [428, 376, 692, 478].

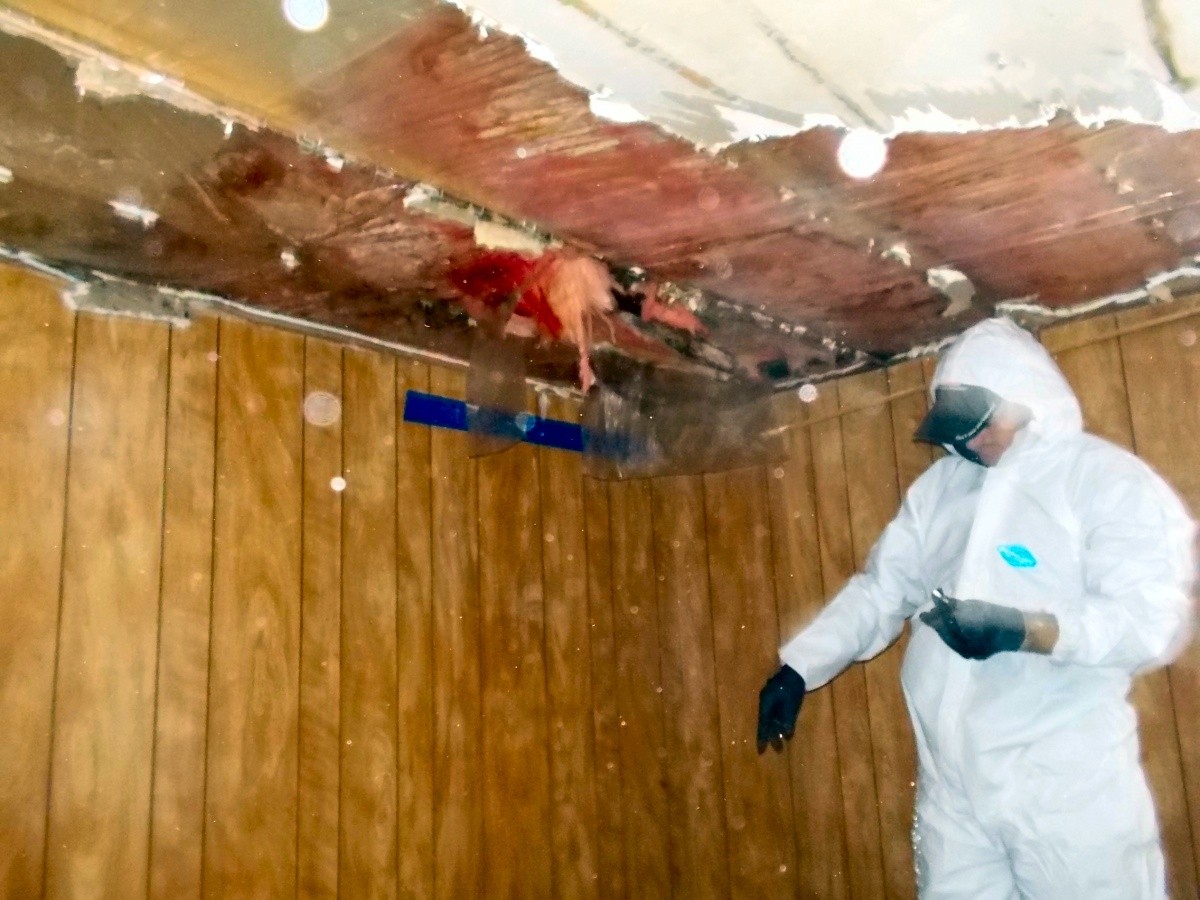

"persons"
[756, 313, 1200, 900]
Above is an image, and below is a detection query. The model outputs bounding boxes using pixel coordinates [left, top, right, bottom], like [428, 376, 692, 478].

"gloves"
[756, 665, 806, 756]
[919, 598, 1025, 661]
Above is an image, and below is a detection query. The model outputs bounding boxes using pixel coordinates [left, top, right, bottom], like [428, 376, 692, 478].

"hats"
[911, 383, 1000, 444]
